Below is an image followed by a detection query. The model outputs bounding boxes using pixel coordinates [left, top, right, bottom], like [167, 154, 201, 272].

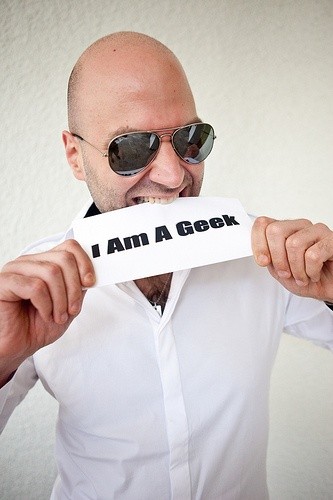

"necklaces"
[148, 273, 172, 317]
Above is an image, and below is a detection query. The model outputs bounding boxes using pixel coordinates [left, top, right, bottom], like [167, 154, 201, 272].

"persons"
[0, 32, 333, 500]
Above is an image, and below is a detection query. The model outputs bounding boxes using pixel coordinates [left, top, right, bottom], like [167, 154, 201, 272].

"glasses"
[68, 122, 218, 178]
